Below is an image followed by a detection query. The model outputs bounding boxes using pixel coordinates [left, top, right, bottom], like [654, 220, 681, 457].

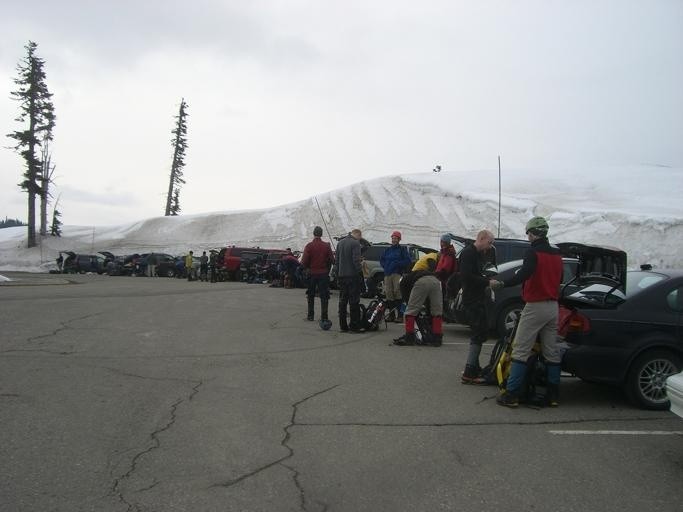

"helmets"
[441, 235, 451, 243]
[391, 231, 401, 239]
[526, 216, 549, 234]
[319, 319, 332, 330]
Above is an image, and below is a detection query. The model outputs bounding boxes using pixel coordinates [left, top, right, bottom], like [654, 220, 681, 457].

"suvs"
[57, 250, 230, 279]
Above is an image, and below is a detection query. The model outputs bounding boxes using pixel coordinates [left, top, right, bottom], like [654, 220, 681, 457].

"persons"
[495, 216, 564, 408]
[185, 225, 456, 347]
[56, 254, 63, 274]
[458, 230, 503, 386]
[146, 252, 157, 276]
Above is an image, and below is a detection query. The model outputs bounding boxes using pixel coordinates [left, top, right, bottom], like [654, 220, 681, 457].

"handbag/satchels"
[415, 307, 432, 344]
[362, 301, 385, 331]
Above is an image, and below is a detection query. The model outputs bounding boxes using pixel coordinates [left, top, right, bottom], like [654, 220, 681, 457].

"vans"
[216, 245, 299, 281]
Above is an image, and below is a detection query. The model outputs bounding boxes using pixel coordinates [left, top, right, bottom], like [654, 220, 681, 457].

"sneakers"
[393, 334, 418, 345]
[544, 396, 560, 405]
[341, 324, 366, 333]
[496, 394, 519, 406]
[461, 375, 488, 386]
[424, 335, 441, 346]
